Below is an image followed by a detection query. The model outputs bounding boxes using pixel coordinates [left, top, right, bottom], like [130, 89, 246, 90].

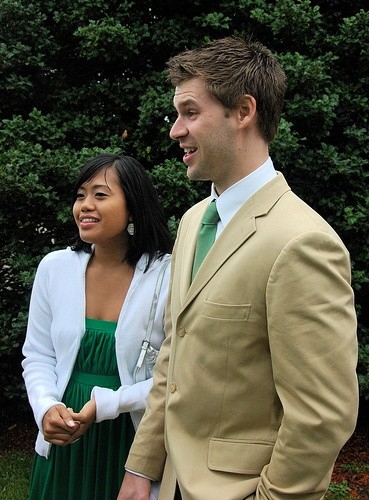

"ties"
[191, 198, 220, 285]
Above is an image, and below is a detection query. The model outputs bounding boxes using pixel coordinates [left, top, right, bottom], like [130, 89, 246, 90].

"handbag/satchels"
[131, 256, 175, 385]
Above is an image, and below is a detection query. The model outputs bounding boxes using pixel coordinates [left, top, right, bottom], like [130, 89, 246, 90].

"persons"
[22, 154, 173, 500]
[116, 36, 359, 500]
